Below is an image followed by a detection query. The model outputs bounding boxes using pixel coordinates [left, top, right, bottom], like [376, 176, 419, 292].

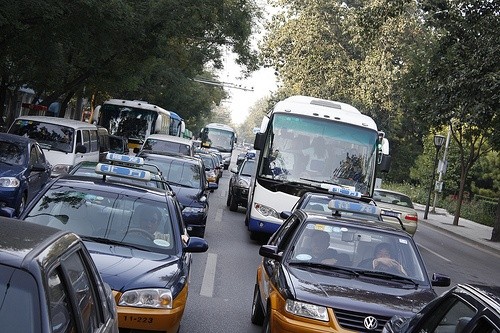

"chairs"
[46, 195, 96, 238]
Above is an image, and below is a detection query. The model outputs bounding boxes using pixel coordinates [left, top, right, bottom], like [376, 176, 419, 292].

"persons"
[296, 230, 342, 266]
[124, 206, 171, 249]
[356, 243, 408, 276]
[277, 129, 364, 183]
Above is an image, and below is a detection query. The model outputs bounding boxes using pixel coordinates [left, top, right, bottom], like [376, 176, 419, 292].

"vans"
[7, 116, 100, 178]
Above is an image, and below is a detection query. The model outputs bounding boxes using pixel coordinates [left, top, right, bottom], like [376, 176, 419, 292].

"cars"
[0, 215, 119, 333]
[381, 282, 500, 333]
[280, 185, 384, 224]
[0, 162, 209, 333]
[369, 188, 419, 237]
[0, 133, 52, 219]
[66, 97, 256, 240]
[251, 199, 452, 333]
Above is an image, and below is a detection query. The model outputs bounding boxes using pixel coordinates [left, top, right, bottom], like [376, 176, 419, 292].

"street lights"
[423, 135, 446, 220]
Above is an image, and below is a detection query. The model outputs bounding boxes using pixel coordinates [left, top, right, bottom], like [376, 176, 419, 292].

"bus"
[245, 95, 389, 241]
